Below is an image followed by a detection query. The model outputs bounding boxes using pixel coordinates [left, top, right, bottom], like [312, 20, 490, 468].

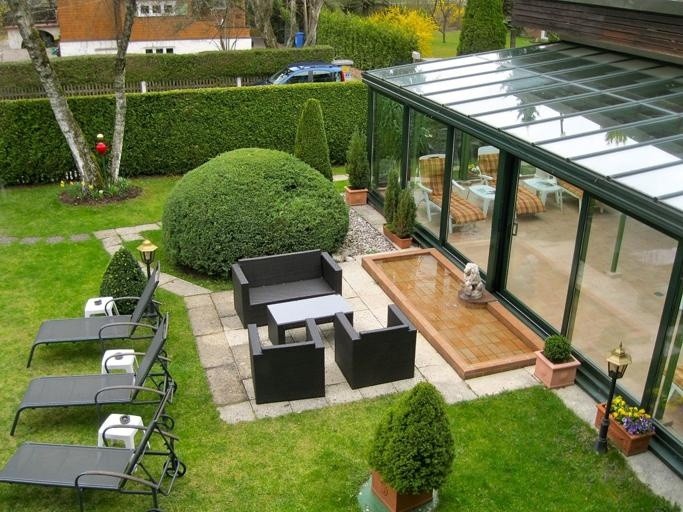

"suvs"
[246, 62, 343, 86]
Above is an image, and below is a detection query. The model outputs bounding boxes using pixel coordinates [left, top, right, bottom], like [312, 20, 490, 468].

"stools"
[101, 349, 139, 374]
[85, 297, 119, 317]
[97, 413, 150, 473]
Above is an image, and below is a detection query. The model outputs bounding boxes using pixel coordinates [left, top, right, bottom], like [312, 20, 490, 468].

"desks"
[468, 185, 517, 222]
[267, 294, 354, 345]
[521, 178, 564, 211]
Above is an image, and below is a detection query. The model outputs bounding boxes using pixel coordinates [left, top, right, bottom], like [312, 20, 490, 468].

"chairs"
[334, 304, 417, 389]
[27, 260, 163, 367]
[478, 145, 546, 220]
[246, 318, 325, 405]
[418, 153, 486, 234]
[10, 312, 177, 436]
[535, 168, 604, 214]
[0, 379, 186, 512]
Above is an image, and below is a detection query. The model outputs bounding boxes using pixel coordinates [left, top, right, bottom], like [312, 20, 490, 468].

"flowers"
[605, 394, 655, 435]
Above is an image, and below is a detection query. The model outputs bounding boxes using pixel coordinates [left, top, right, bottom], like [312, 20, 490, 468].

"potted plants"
[383, 168, 417, 249]
[532, 334, 581, 389]
[367, 382, 455, 512]
[344, 124, 370, 205]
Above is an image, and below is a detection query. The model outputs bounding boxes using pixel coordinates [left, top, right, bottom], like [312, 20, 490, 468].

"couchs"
[232, 249, 342, 328]
